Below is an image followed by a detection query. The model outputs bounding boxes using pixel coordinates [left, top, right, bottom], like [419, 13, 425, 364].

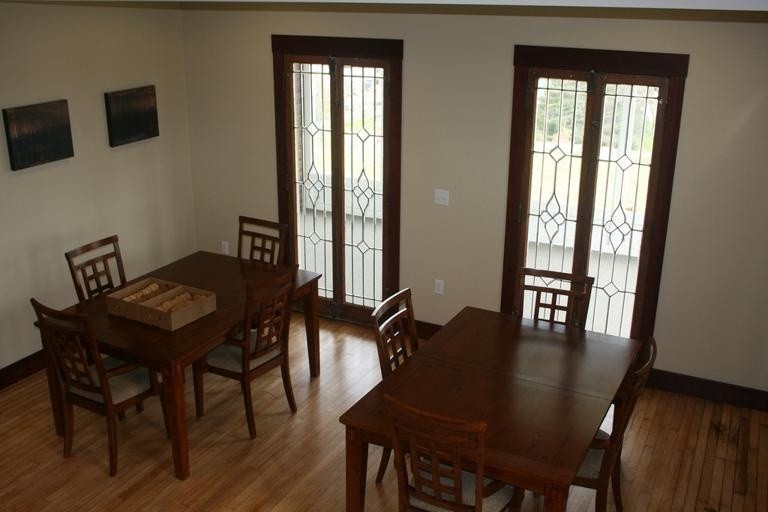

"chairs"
[29, 296, 170, 476]
[191, 264, 299, 441]
[511, 266, 597, 334]
[235, 214, 289, 270]
[368, 287, 421, 489]
[383, 395, 489, 512]
[570, 335, 658, 511]
[64, 234, 147, 412]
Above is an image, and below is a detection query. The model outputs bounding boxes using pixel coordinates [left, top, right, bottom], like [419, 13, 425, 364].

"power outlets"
[432, 278, 445, 297]
[219, 241, 230, 256]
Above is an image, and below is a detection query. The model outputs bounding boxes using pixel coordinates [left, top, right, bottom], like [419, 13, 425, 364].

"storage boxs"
[105, 277, 216, 333]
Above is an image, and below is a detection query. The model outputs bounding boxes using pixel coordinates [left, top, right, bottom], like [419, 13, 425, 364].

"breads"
[122, 283, 207, 314]
